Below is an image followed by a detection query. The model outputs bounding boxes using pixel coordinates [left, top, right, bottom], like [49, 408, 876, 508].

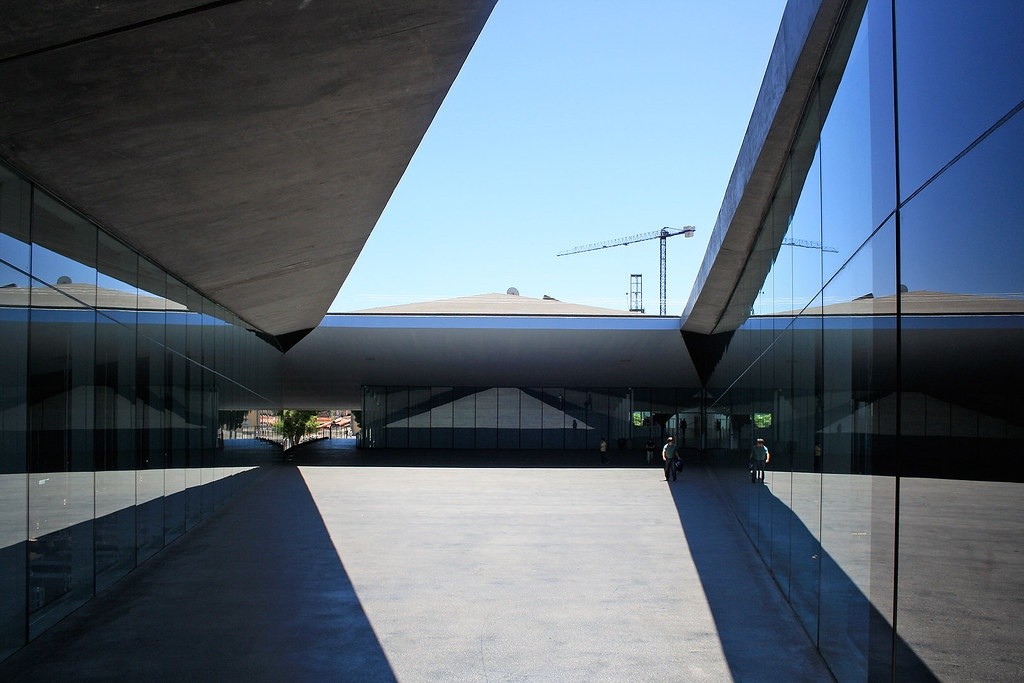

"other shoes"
[761, 480, 764, 483]
[666, 477, 668, 481]
[752, 478, 756, 482]
[673, 477, 676, 480]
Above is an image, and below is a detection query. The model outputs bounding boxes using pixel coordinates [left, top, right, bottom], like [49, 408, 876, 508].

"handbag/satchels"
[744, 463, 752, 475]
[674, 460, 683, 473]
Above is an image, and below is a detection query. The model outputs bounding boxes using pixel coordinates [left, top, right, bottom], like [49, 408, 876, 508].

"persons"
[662, 437, 682, 482]
[349, 429, 353, 438]
[599, 437, 609, 464]
[646, 439, 655, 464]
[679, 417, 686, 435]
[749, 438, 769, 484]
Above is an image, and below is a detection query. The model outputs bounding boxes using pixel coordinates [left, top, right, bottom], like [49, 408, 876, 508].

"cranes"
[554, 223, 696, 316]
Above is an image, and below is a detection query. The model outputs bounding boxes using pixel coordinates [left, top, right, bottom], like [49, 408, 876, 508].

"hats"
[667, 437, 673, 442]
[757, 439, 765, 442]
